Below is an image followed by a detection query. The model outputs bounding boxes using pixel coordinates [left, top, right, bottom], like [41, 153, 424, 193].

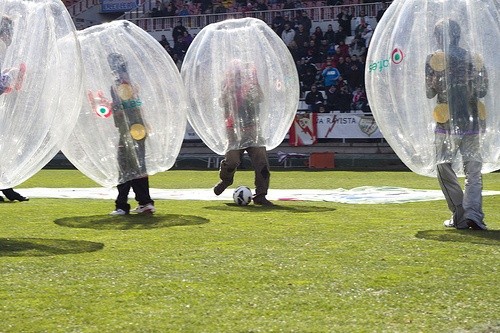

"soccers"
[233, 186, 252, 206]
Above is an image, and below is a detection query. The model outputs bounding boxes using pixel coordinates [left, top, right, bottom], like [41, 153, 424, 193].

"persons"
[425, 18, 489, 232]
[214, 59, 275, 208]
[108, 51, 156, 218]
[267, 0, 388, 114]
[0, 15, 29, 203]
[140, 0, 267, 30]
[159, 20, 197, 72]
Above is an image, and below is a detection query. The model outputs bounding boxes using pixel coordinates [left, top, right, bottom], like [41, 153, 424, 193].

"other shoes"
[444, 219, 459, 228]
[14, 194, 29, 201]
[467, 217, 487, 230]
[214, 181, 229, 195]
[253, 194, 274, 206]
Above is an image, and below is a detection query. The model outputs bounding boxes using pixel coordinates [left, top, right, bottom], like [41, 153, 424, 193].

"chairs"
[147, 17, 376, 113]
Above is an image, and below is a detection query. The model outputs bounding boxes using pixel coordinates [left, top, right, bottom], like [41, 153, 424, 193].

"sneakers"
[109, 209, 128, 216]
[130, 202, 156, 213]
